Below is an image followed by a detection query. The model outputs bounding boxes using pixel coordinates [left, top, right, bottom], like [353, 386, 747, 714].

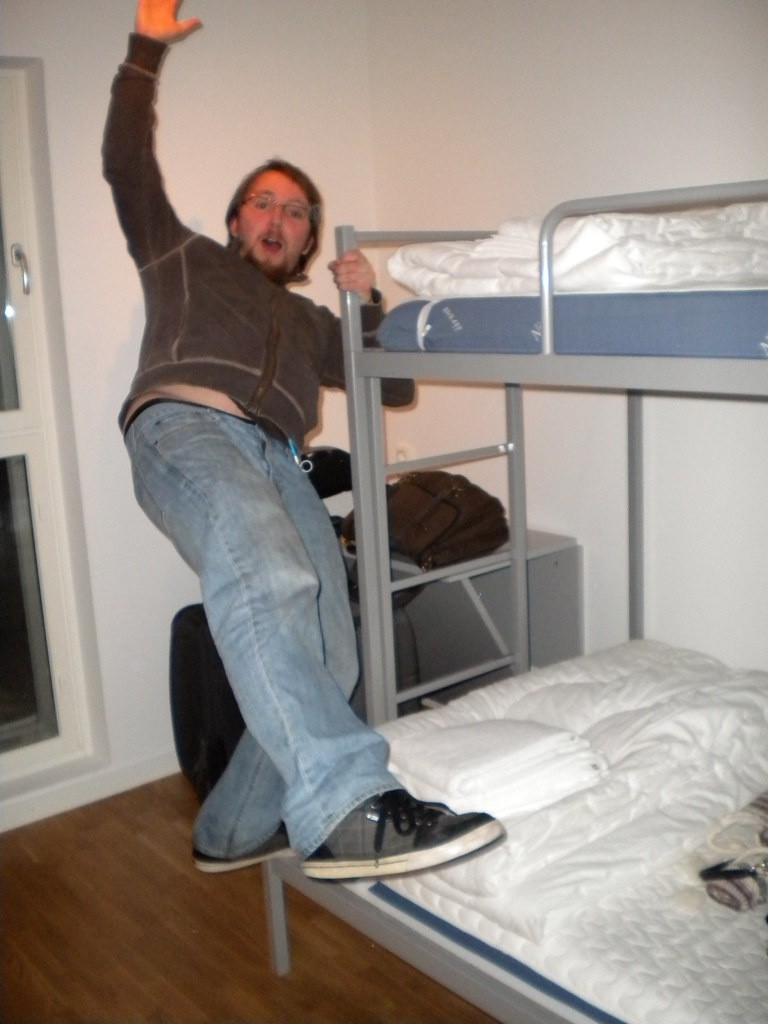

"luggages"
[172, 603, 247, 805]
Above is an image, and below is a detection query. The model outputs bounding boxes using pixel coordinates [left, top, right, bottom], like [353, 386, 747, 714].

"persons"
[99, 1, 506, 881]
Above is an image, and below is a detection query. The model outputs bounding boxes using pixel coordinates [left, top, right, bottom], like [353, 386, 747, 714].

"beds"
[277, 636, 768, 1024]
[362, 290, 768, 366]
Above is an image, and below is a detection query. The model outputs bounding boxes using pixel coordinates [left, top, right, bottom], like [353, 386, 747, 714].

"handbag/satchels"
[341, 470, 510, 571]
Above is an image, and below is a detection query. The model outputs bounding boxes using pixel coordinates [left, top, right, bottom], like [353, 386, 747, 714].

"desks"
[328, 527, 578, 715]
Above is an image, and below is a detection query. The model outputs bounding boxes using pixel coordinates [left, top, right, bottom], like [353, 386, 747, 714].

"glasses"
[238, 193, 315, 222]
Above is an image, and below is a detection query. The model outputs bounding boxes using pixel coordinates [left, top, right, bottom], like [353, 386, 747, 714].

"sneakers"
[193, 819, 297, 873]
[300, 790, 505, 878]
